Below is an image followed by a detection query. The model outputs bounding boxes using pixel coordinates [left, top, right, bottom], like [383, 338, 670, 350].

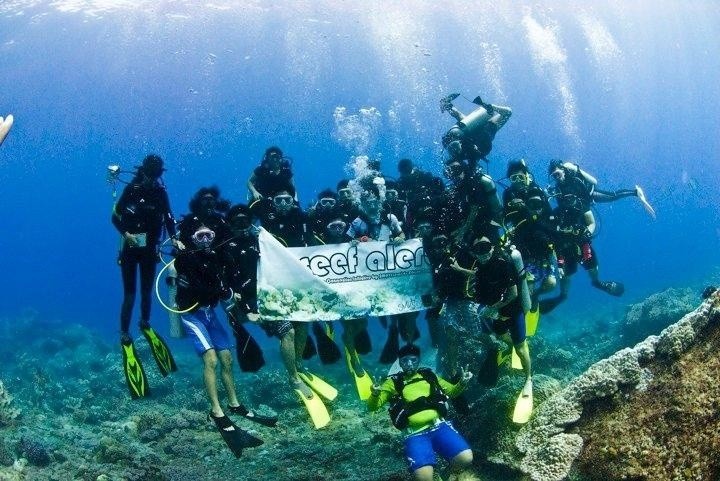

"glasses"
[418, 223, 434, 233]
[200, 196, 216, 208]
[510, 174, 526, 183]
[472, 242, 493, 255]
[274, 195, 293, 206]
[327, 222, 346, 230]
[337, 187, 354, 198]
[320, 198, 336, 207]
[232, 214, 251, 226]
[400, 356, 419, 366]
[193, 230, 216, 242]
[444, 165, 463, 179]
[549, 169, 564, 179]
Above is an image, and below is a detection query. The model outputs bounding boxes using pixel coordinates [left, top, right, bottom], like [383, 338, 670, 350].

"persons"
[112, 154, 186, 401]
